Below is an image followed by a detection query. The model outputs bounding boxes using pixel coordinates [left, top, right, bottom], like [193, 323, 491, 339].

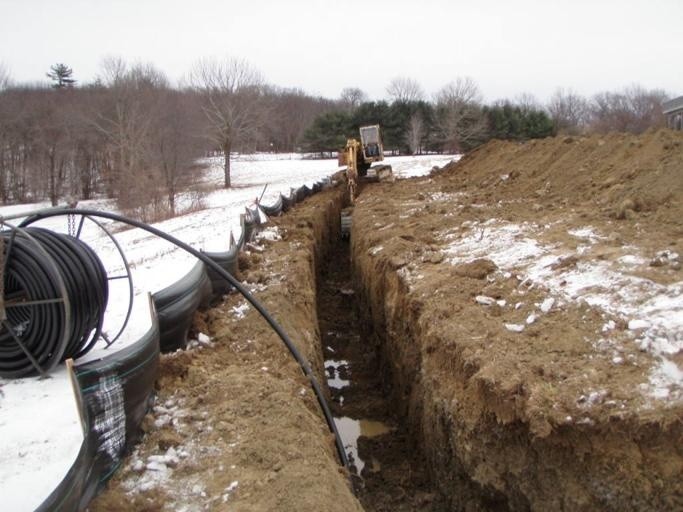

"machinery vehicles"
[336, 120, 393, 241]
